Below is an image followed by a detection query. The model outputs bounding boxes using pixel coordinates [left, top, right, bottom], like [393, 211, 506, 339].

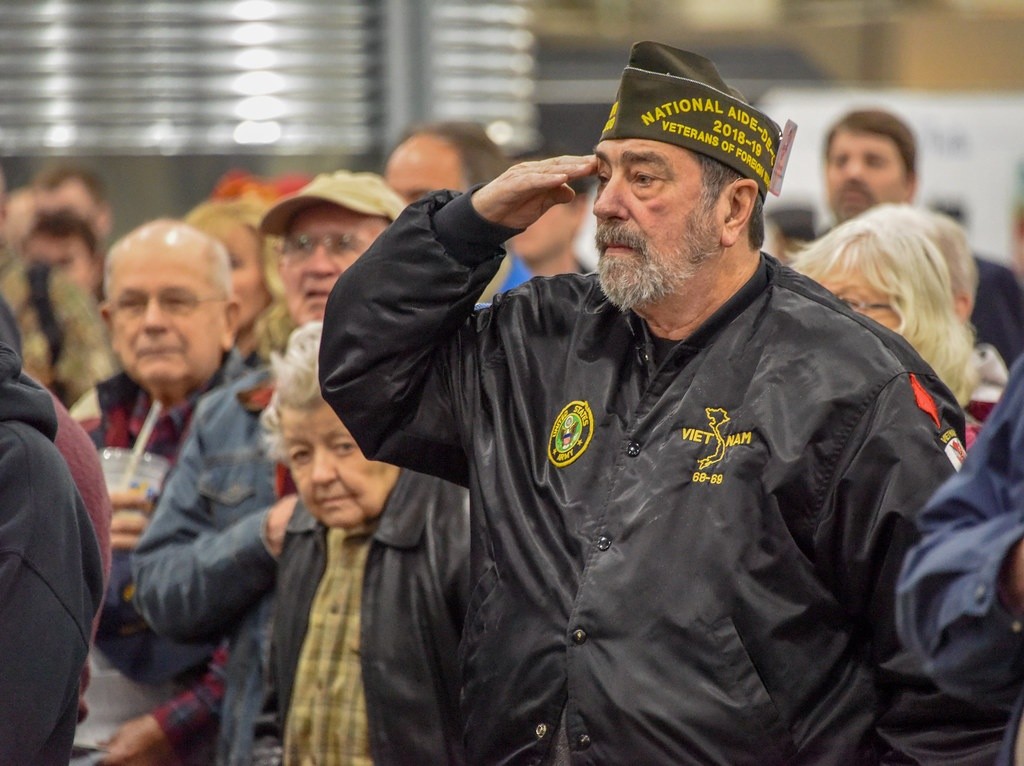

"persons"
[253, 321, 475, 765]
[0, 170, 123, 414]
[784, 216, 987, 461]
[892, 359, 1024, 765]
[383, 122, 535, 316]
[132, 172, 419, 765]
[51, 383, 113, 725]
[70, 217, 259, 765]
[320, 38, 971, 766]
[767, 203, 819, 264]
[189, 204, 304, 373]
[8, 165, 118, 301]
[859, 201, 1008, 423]
[820, 110, 1023, 366]
[0, 301, 102, 766]
[499, 143, 596, 280]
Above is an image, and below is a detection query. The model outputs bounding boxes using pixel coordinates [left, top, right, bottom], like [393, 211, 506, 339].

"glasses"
[841, 297, 894, 315]
[100, 286, 230, 319]
[273, 230, 369, 264]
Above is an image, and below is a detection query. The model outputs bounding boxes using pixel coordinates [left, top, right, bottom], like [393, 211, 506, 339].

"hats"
[260, 167, 406, 238]
[599, 40, 784, 206]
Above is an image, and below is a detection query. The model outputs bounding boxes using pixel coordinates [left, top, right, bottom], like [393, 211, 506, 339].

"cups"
[100, 446, 169, 520]
